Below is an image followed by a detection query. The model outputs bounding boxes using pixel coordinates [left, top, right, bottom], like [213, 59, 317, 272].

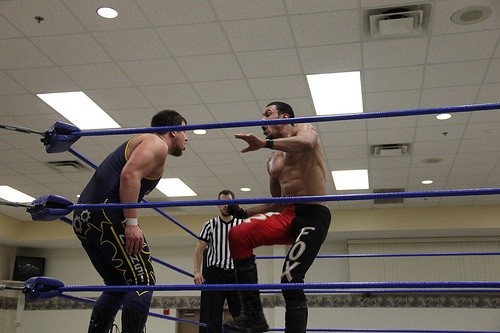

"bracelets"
[194, 271, 201, 274]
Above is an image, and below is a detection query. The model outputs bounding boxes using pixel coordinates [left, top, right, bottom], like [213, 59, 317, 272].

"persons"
[222, 101, 332, 333]
[194, 190, 244, 333]
[72, 109, 189, 333]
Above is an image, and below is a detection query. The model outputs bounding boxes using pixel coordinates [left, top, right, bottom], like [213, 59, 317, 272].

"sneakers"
[221, 314, 269, 333]
